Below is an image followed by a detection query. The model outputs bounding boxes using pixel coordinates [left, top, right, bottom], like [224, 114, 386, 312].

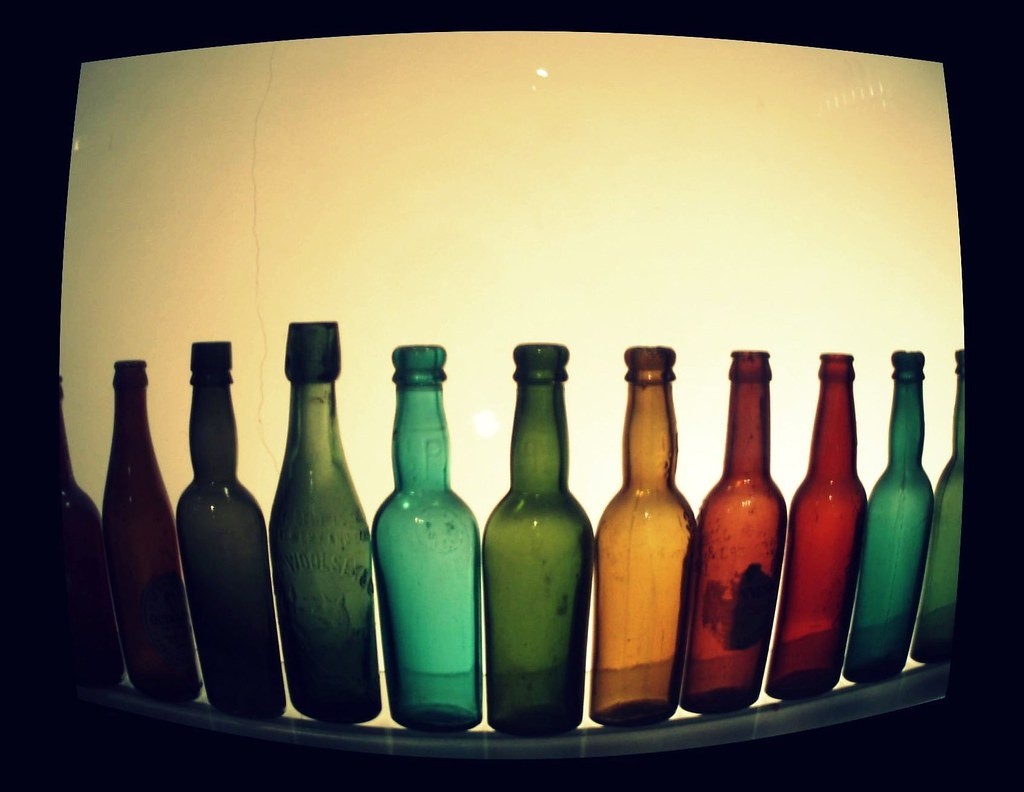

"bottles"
[772, 355, 868, 703]
[591, 347, 695, 729]
[911, 350, 966, 663]
[372, 344, 479, 737]
[268, 323, 383, 727]
[173, 342, 287, 719]
[57, 379, 127, 691]
[480, 344, 592, 734]
[103, 360, 202, 711]
[680, 350, 788, 715]
[842, 353, 935, 686]
